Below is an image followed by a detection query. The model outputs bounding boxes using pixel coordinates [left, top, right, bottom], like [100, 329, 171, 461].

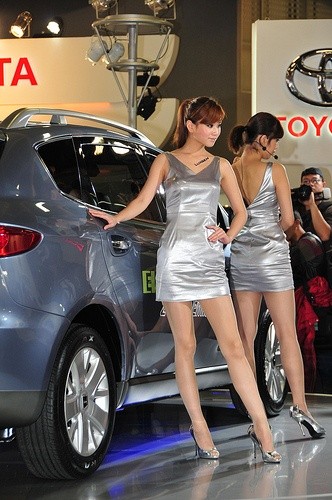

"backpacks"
[296, 234, 332, 337]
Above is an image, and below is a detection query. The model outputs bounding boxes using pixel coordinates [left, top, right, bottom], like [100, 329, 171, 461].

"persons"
[89, 96, 283, 464]
[276, 210, 323, 278]
[230, 112, 325, 438]
[296, 167, 331, 242]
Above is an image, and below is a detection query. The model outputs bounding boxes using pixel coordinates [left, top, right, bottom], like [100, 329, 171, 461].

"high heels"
[248, 424, 281, 463]
[189, 424, 221, 459]
[288, 405, 326, 438]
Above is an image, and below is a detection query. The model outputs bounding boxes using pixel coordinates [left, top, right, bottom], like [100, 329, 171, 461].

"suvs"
[0, 105, 292, 480]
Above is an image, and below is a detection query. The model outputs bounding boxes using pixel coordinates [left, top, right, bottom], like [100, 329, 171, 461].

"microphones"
[261, 146, 278, 159]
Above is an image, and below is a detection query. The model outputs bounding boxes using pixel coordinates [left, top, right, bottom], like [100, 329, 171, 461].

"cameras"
[293, 184, 312, 202]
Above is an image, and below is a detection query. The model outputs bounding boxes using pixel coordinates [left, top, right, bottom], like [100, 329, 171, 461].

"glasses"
[301, 179, 322, 185]
